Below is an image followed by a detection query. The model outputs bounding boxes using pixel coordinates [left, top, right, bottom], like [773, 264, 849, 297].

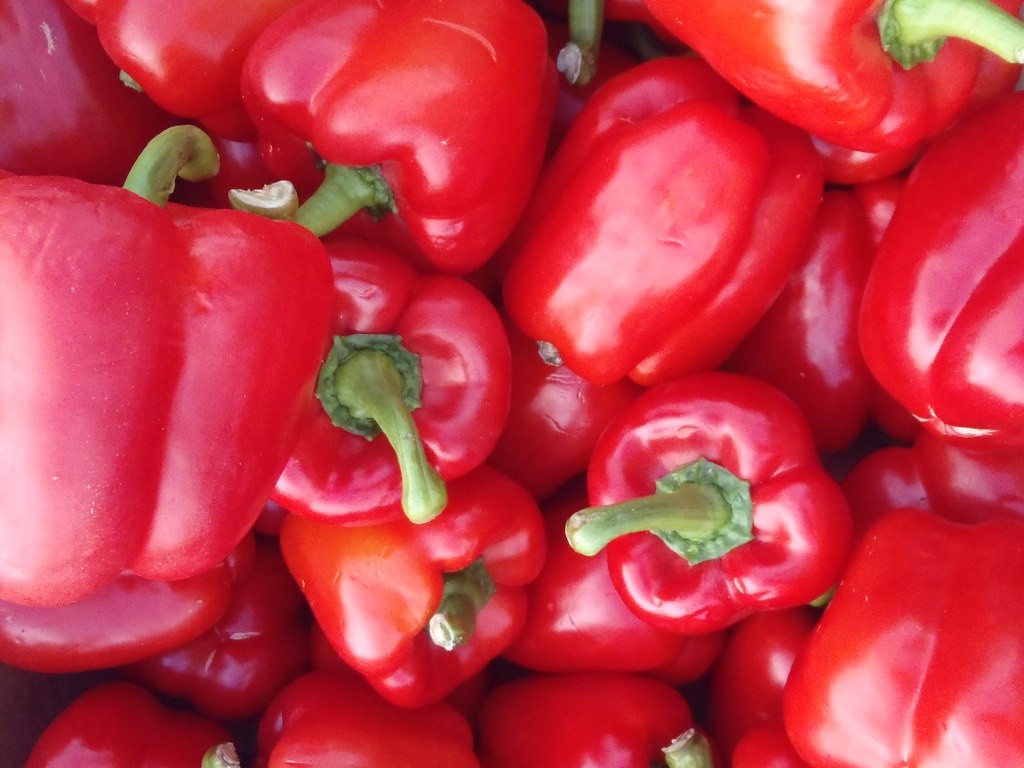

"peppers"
[0, 0, 1024, 768]
